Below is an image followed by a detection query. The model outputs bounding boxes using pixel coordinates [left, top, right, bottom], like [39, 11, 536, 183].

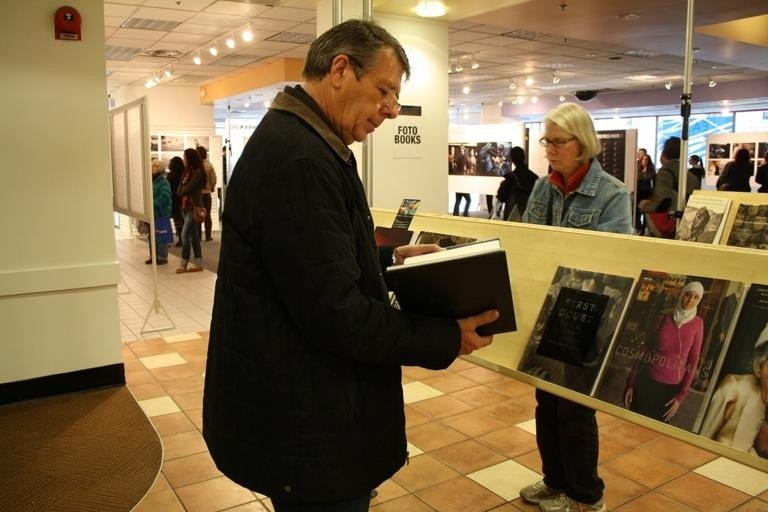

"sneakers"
[185, 266, 203, 272]
[145, 259, 152, 264]
[176, 265, 186, 273]
[176, 241, 182, 246]
[157, 260, 168, 265]
[519, 480, 607, 512]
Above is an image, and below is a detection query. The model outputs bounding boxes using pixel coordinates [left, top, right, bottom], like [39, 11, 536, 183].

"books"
[384, 235, 519, 336]
[513, 264, 768, 458]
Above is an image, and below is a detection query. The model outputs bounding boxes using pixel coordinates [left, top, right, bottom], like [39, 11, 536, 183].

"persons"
[449, 187, 472, 217]
[144, 147, 229, 274]
[726, 205, 768, 251]
[484, 194, 499, 217]
[717, 148, 754, 192]
[497, 146, 538, 221]
[202, 20, 501, 512]
[521, 102, 633, 235]
[756, 151, 768, 194]
[449, 145, 509, 176]
[708, 161, 719, 176]
[635, 136, 706, 238]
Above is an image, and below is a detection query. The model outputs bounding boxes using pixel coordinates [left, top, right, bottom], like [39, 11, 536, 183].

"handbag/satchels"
[507, 203, 522, 222]
[155, 215, 174, 243]
[645, 185, 689, 237]
[193, 206, 207, 224]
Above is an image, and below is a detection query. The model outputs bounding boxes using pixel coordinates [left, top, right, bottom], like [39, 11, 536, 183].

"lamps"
[498, 72, 717, 108]
[144, 21, 255, 89]
[416, 0, 447, 17]
[448, 51, 481, 74]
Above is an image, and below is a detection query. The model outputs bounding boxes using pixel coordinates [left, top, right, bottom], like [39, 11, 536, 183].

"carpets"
[0, 384, 164, 512]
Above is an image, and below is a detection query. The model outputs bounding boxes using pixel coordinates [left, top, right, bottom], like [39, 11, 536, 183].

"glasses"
[329, 53, 402, 114]
[539, 136, 576, 149]
